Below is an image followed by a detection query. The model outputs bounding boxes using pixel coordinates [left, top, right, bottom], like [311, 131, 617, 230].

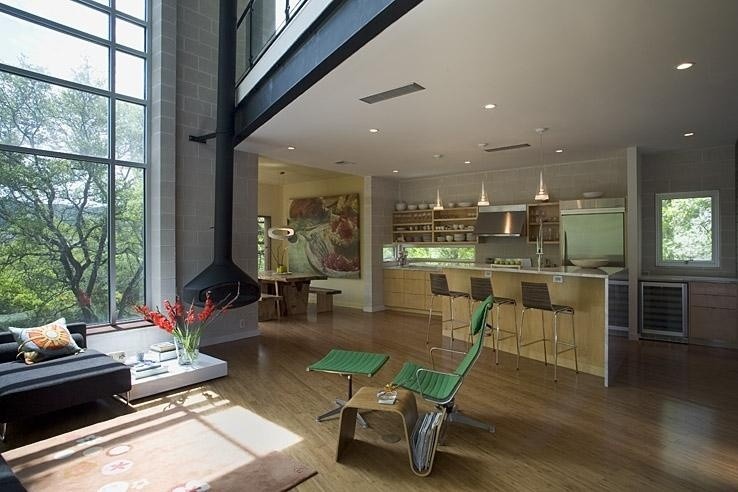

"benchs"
[308, 286, 342, 314]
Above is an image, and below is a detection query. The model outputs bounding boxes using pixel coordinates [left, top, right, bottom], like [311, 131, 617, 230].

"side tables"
[116, 348, 227, 403]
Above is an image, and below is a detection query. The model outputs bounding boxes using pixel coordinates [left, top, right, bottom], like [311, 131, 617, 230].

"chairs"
[426, 271, 580, 383]
[393, 293, 498, 448]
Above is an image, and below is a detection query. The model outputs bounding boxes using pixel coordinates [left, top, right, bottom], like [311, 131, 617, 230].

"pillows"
[7, 316, 87, 366]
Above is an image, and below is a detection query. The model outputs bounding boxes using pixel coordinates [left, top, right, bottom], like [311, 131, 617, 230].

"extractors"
[473, 204, 526, 238]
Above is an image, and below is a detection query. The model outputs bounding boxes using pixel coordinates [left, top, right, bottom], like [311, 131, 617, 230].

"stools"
[305, 347, 392, 431]
[258, 292, 285, 323]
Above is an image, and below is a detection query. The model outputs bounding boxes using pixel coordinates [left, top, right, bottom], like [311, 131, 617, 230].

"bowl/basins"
[394, 203, 436, 210]
[447, 201, 454, 208]
[409, 225, 432, 230]
[434, 224, 474, 230]
[493, 260, 521, 265]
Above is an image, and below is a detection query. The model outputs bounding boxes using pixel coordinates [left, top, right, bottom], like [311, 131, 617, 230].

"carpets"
[0, 401, 317, 491]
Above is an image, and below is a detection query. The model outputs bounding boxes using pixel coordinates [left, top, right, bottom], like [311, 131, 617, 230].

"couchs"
[0, 322, 134, 441]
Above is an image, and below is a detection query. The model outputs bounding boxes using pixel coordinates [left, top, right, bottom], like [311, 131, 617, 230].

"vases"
[175, 332, 200, 365]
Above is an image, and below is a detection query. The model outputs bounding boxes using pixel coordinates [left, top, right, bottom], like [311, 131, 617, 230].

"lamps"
[478, 141, 491, 209]
[430, 154, 446, 211]
[533, 127, 551, 202]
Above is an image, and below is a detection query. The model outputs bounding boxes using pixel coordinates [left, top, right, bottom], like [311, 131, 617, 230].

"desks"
[336, 384, 450, 476]
[259, 269, 328, 316]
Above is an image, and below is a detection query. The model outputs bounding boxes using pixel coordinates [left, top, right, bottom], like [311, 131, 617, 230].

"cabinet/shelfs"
[390, 209, 433, 244]
[382, 269, 442, 314]
[433, 206, 482, 246]
[526, 201, 559, 246]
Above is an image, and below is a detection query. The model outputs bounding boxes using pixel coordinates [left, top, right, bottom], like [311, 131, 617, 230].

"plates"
[457, 202, 474, 207]
[436, 233, 476, 242]
[397, 234, 431, 242]
[396, 227, 407, 230]
[582, 192, 604, 198]
[568, 259, 609, 268]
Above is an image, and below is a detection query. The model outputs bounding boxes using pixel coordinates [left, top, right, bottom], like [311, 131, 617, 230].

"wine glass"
[393, 212, 432, 223]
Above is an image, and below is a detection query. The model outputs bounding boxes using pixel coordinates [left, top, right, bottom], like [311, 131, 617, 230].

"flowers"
[132, 281, 243, 366]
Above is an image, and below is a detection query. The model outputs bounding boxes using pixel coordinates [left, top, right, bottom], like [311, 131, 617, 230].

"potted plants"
[266, 243, 300, 272]
